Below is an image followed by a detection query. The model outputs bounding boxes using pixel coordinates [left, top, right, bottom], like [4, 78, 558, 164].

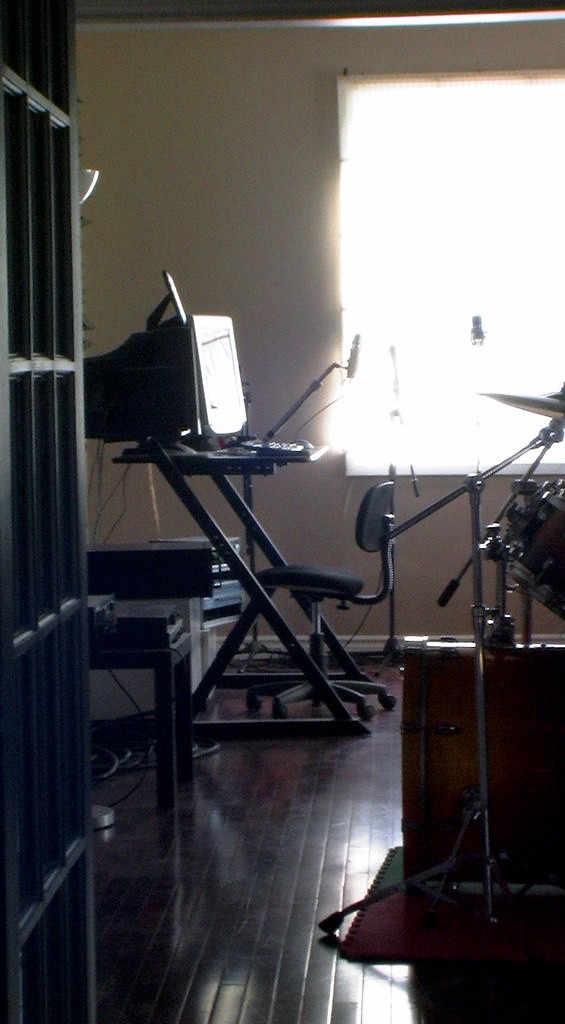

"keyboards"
[241, 440, 305, 456]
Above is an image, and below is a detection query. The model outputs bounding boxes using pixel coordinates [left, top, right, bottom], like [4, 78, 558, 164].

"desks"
[90, 631, 193, 812]
[113, 445, 370, 738]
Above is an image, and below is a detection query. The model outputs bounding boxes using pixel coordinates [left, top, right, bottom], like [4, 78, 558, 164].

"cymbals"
[474, 386, 565, 422]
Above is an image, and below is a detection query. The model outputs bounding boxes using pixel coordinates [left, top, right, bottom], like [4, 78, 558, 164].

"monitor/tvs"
[83, 314, 247, 446]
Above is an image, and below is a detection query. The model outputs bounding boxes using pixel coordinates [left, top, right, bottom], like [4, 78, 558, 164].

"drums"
[503, 477, 565, 619]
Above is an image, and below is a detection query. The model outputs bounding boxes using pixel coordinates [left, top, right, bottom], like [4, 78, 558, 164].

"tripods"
[322, 414, 565, 940]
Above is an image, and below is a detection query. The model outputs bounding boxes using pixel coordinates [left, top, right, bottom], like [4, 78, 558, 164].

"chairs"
[245, 482, 397, 721]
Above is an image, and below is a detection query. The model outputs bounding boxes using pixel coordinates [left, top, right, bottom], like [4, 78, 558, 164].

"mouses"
[290, 440, 314, 449]
